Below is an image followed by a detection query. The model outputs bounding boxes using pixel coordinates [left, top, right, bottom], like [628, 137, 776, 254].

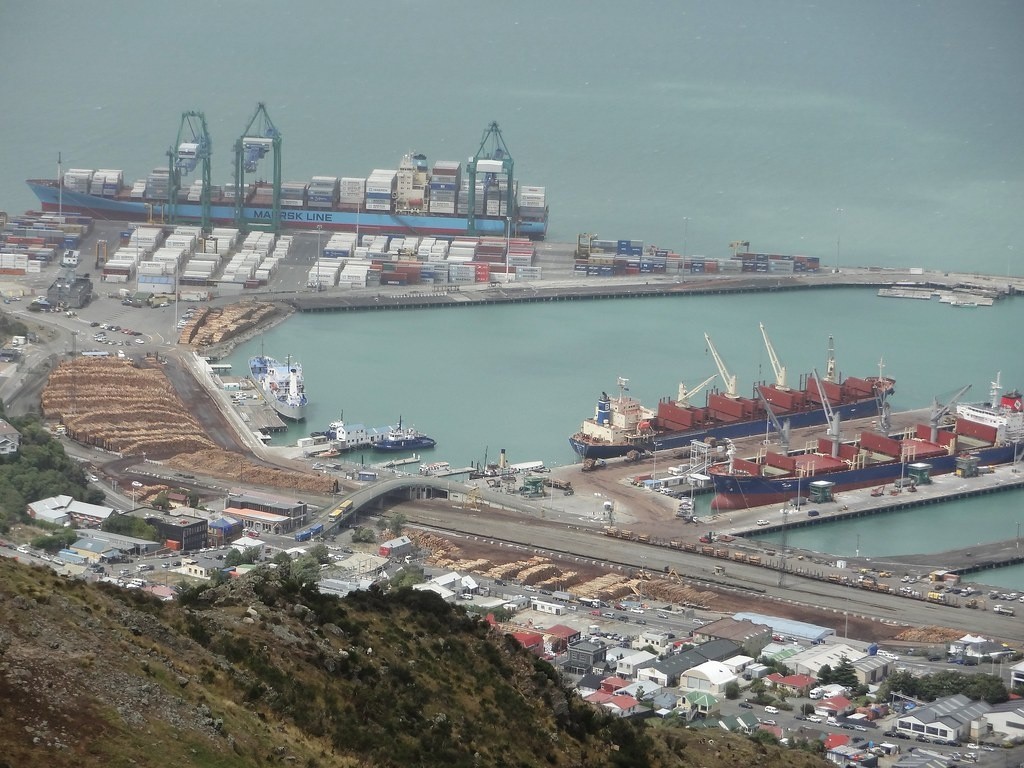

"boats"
[703, 370, 1024, 509]
[368, 413, 438, 452]
[247, 329, 308, 420]
[469, 448, 546, 480]
[566, 322, 899, 462]
[24, 97, 550, 242]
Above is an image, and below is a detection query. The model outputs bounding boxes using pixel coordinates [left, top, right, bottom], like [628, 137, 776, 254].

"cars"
[0, 497, 354, 591]
[756, 519, 770, 525]
[459, 568, 1024, 762]
[2, 294, 198, 358]
